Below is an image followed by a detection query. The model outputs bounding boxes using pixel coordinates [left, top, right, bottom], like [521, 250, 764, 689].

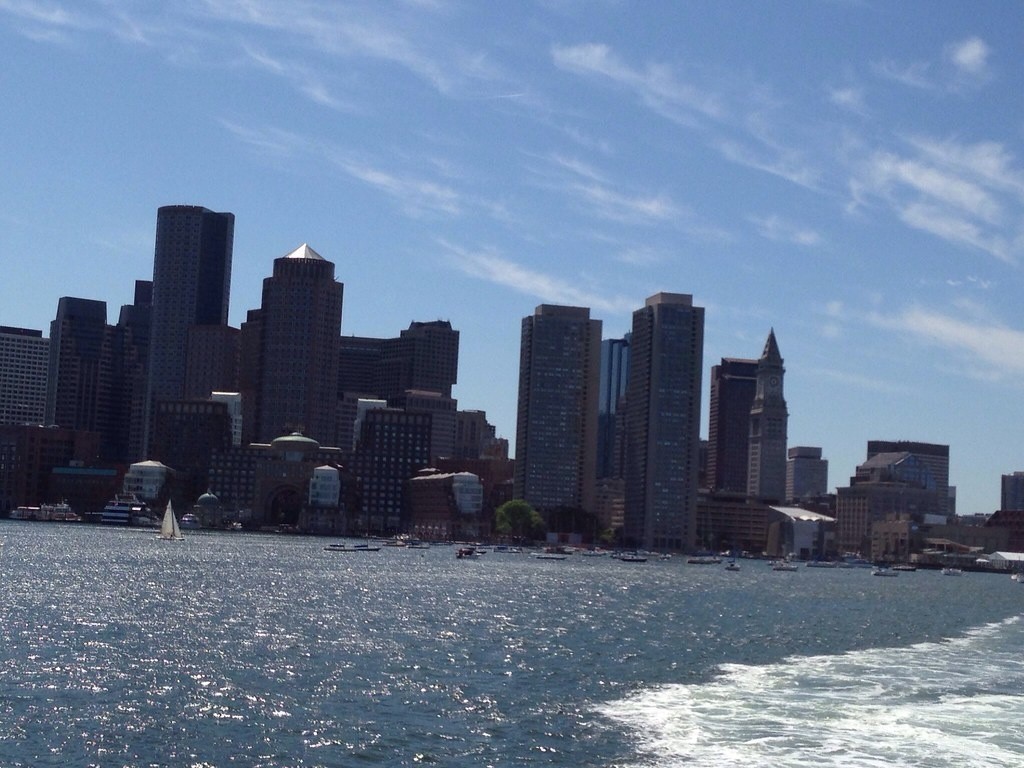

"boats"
[457, 542, 963, 577]
[8, 502, 84, 523]
[324, 543, 382, 552]
[181, 513, 201, 530]
[1011, 572, 1024, 584]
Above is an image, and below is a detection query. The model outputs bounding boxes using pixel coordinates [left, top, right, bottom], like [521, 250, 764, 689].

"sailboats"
[157, 498, 185, 541]
